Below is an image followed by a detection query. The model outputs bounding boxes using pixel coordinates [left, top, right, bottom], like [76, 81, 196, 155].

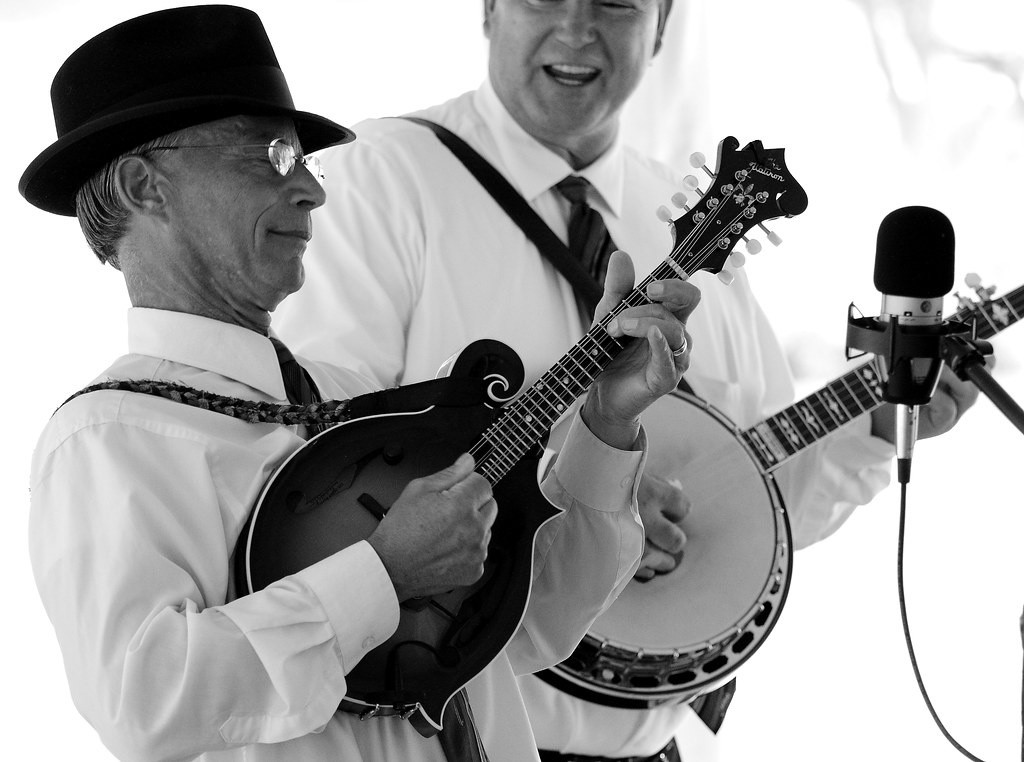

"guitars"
[532, 271, 1023, 713]
[227, 136, 812, 738]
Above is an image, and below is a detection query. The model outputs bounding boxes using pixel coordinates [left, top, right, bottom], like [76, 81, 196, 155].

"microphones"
[875, 206, 956, 483]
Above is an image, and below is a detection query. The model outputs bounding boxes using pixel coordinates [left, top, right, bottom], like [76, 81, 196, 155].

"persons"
[267, 0, 997, 762]
[19, 6, 699, 762]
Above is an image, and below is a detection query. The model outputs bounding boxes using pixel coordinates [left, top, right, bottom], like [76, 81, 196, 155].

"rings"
[672, 338, 687, 356]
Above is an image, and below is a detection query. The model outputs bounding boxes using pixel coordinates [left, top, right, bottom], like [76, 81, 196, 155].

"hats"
[20, 3, 356, 217]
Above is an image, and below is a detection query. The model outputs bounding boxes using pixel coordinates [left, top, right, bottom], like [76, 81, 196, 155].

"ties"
[270, 336, 491, 762]
[555, 175, 621, 347]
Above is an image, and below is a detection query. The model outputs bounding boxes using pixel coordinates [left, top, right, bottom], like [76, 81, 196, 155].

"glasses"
[126, 139, 326, 187]
